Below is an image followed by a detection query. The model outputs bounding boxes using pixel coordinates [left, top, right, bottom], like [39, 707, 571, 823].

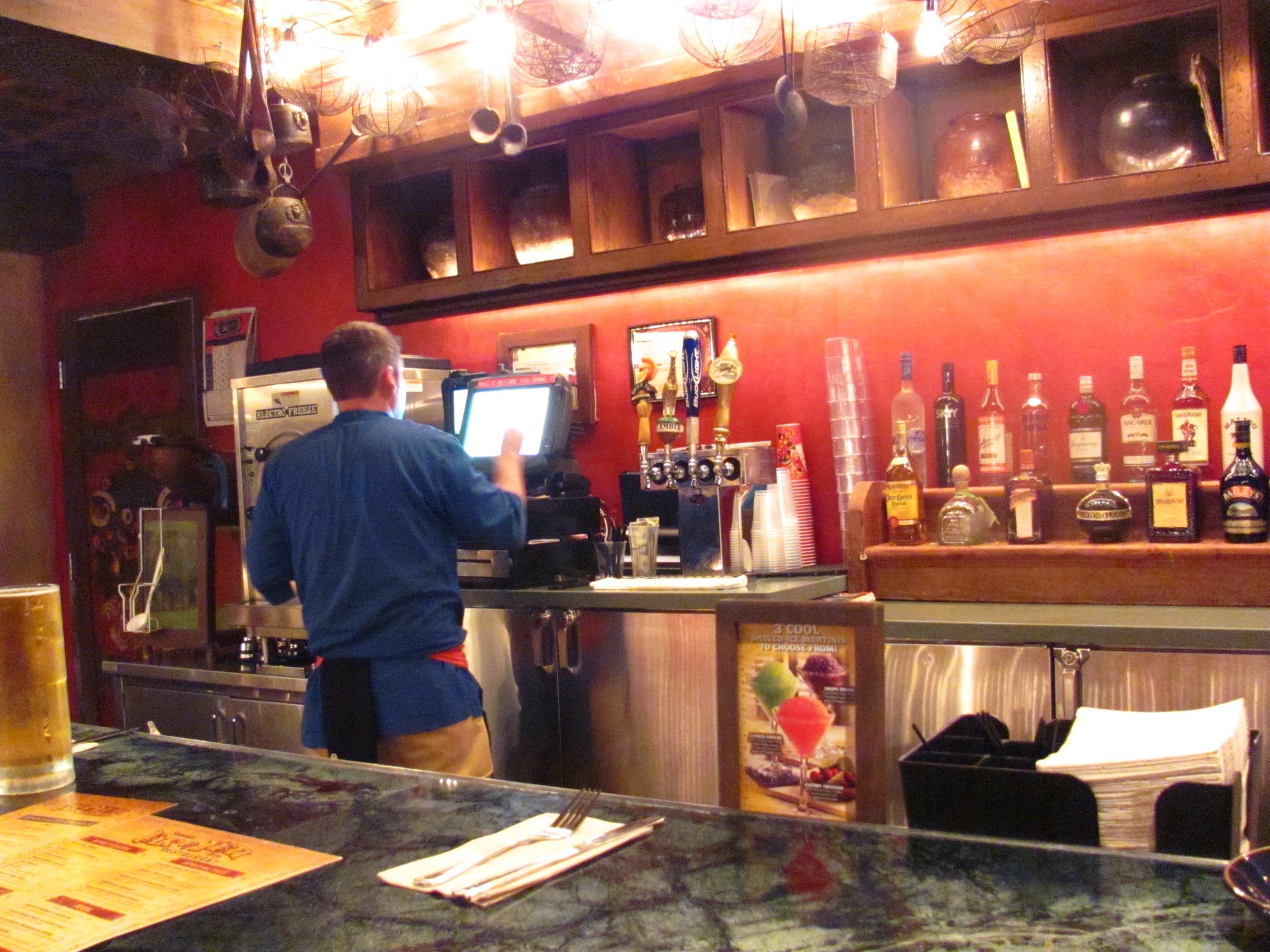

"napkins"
[374, 782, 656, 911]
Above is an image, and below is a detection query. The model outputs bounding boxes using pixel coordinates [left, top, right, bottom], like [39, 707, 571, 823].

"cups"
[728, 422, 817, 577]
[593, 541, 627, 579]
[627, 525, 660, 578]
[0, 581, 77, 797]
[825, 337, 876, 565]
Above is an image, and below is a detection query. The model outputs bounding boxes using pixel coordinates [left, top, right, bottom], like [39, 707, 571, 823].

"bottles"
[1220, 344, 1264, 473]
[1068, 375, 1107, 484]
[1145, 455, 1199, 543]
[973, 359, 1013, 486]
[884, 421, 927, 546]
[416, 182, 706, 283]
[937, 464, 990, 546]
[1219, 420, 1270, 544]
[788, 70, 1203, 220]
[1017, 372, 1051, 478]
[1076, 462, 1134, 544]
[890, 351, 926, 488]
[1005, 449, 1054, 543]
[1119, 355, 1157, 483]
[932, 361, 967, 488]
[1170, 347, 1213, 481]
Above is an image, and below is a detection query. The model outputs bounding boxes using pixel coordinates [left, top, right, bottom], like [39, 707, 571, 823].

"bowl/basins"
[1222, 844, 1270, 916]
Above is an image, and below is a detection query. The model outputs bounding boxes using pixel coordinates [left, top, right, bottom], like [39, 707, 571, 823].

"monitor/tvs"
[457, 377, 572, 464]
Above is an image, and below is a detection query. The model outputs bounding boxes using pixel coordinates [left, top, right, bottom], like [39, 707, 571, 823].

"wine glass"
[745, 666, 850, 819]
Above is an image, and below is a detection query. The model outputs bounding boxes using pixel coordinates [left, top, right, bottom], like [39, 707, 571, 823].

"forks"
[413, 786, 601, 892]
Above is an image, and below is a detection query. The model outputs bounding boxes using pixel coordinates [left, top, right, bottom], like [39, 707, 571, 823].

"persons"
[246, 319, 527, 779]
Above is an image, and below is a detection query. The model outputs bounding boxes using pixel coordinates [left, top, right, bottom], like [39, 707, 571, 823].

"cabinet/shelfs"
[313, 3, 1270, 335]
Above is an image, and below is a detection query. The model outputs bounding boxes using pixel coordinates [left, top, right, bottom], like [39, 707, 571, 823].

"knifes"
[450, 812, 667, 900]
